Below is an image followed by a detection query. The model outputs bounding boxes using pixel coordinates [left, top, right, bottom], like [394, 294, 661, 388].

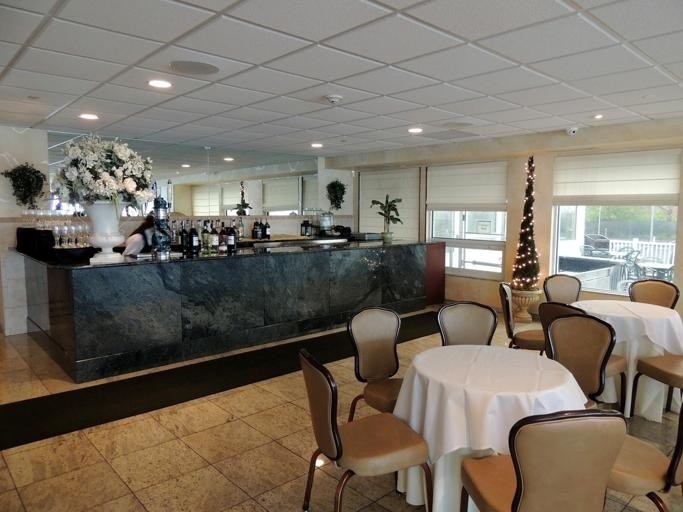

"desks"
[390, 344, 588, 512]
[568, 299, 683, 424]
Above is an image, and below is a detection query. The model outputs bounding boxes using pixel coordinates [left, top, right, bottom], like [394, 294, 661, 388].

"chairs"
[606, 247, 676, 292]
[603, 405, 683, 511]
[460, 409, 628, 512]
[627, 279, 680, 310]
[630, 354, 683, 417]
[347, 306, 404, 423]
[437, 303, 498, 346]
[298, 347, 434, 512]
[499, 274, 626, 414]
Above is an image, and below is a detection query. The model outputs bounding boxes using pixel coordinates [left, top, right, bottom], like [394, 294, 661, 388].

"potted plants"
[153, 196, 168, 220]
[319, 178, 348, 231]
[370, 194, 403, 244]
[507, 155, 543, 323]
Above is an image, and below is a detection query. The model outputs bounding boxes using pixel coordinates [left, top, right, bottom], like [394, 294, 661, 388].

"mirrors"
[47, 128, 319, 217]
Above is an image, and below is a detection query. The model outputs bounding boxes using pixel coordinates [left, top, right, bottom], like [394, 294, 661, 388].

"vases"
[75, 200, 132, 267]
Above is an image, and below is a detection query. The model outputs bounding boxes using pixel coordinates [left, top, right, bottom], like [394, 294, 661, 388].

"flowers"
[50, 132, 158, 212]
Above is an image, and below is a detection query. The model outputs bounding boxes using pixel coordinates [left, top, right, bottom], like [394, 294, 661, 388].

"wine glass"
[22, 210, 90, 248]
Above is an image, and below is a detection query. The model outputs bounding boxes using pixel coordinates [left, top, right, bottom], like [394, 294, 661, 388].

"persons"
[122, 211, 169, 257]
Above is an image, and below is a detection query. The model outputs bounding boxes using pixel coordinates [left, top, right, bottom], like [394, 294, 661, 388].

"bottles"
[171, 217, 244, 257]
[251, 218, 270, 239]
[300, 212, 334, 236]
